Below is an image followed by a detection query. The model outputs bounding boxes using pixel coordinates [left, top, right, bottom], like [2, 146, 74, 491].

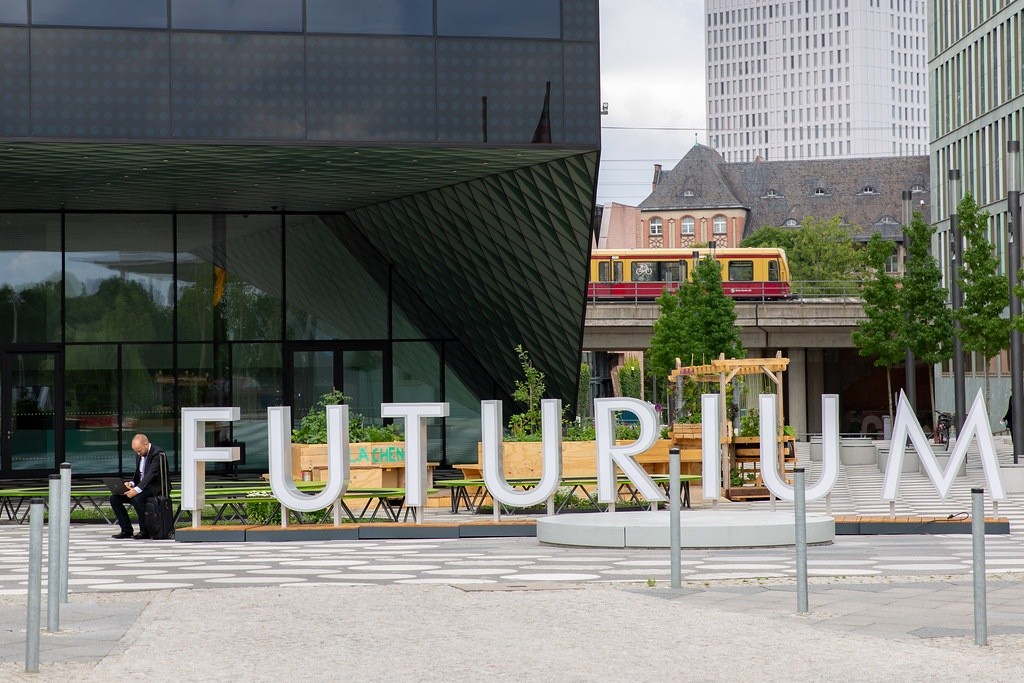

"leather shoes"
[133, 531, 151, 539]
[112, 532, 134, 539]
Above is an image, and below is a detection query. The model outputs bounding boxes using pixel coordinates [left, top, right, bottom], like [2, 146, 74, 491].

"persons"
[109, 433, 172, 540]
[1001, 388, 1015, 456]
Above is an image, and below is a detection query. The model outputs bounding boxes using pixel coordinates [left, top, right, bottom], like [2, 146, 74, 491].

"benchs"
[453, 464, 484, 493]
[0, 476, 703, 526]
[313, 461, 440, 488]
[634, 449, 702, 487]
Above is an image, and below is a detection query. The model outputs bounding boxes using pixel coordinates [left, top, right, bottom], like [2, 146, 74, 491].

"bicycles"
[928, 410, 955, 452]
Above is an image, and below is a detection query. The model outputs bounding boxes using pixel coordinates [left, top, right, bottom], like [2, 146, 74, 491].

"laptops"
[103, 478, 129, 495]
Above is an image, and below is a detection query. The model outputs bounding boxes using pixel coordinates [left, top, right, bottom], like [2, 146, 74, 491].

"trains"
[587, 245, 792, 301]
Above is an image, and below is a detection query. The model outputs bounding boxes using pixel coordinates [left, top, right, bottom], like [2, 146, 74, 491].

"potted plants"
[478, 344, 667, 494]
[291, 386, 405, 489]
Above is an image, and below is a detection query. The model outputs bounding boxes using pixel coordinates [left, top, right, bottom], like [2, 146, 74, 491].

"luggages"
[144, 453, 175, 539]
